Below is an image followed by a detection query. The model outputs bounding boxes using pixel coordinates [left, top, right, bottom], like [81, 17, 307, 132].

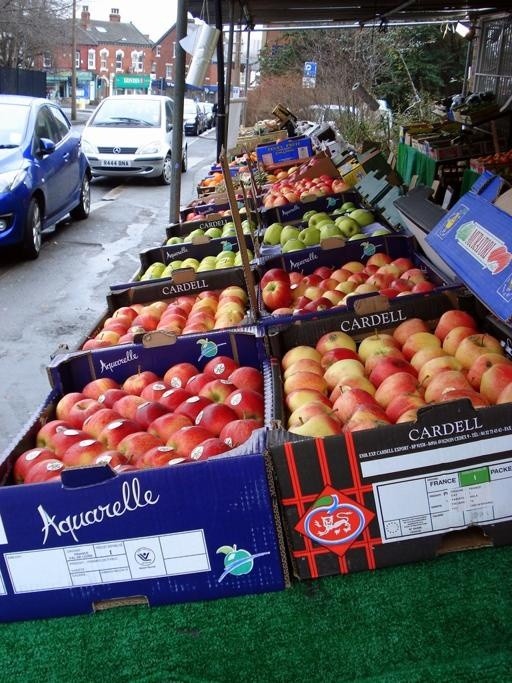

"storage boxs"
[0, 103, 512, 623]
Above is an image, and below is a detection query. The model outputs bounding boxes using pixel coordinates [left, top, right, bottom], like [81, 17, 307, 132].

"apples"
[140, 248, 252, 282]
[166, 219, 256, 246]
[15, 355, 264, 484]
[303, 494, 365, 545]
[261, 252, 434, 315]
[265, 202, 391, 253]
[82, 285, 247, 351]
[281, 309, 512, 485]
[216, 544, 254, 576]
[184, 156, 350, 224]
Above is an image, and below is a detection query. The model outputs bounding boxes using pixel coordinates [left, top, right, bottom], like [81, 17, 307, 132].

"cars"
[0, 95, 91, 259]
[82, 95, 187, 184]
[182, 99, 218, 136]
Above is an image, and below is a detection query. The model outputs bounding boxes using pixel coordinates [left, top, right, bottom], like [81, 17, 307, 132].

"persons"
[40, 86, 62, 106]
[194, 94, 202, 104]
[211, 103, 218, 128]
